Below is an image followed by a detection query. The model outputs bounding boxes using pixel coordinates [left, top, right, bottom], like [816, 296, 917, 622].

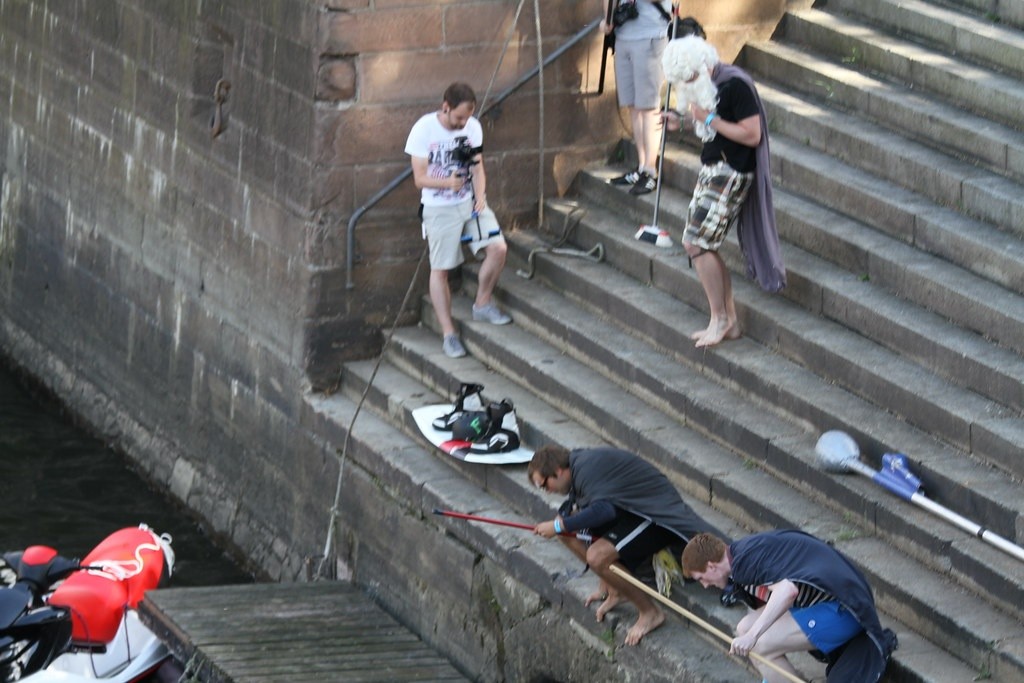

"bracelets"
[706, 112, 714, 125]
[554, 519, 561, 534]
[711, 115, 721, 128]
[680, 115, 684, 132]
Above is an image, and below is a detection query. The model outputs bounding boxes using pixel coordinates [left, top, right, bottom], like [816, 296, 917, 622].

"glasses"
[539, 476, 549, 493]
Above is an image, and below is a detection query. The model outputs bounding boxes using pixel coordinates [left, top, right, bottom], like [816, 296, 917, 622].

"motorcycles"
[0, 527, 180, 683]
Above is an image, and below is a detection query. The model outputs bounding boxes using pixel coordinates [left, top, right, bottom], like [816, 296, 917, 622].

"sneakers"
[611, 169, 641, 185]
[628, 171, 662, 195]
[442, 332, 466, 358]
[472, 304, 512, 325]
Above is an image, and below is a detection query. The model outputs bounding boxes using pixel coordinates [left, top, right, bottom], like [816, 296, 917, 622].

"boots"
[433, 382, 485, 429]
[468, 397, 521, 452]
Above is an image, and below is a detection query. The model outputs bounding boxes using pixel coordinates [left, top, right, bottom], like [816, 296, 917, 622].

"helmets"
[453, 410, 490, 441]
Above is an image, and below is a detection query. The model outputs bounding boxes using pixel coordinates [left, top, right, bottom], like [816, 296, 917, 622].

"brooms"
[635, 1, 675, 249]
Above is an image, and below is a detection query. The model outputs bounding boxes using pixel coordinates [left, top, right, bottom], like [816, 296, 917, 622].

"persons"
[529, 444, 698, 646]
[600, 0, 672, 195]
[403, 83, 512, 359]
[655, 33, 762, 348]
[682, 530, 875, 683]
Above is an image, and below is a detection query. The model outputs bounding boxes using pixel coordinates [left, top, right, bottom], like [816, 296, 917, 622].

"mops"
[431, 508, 684, 599]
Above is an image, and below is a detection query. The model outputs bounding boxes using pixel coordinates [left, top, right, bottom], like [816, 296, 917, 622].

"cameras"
[453, 145, 483, 162]
